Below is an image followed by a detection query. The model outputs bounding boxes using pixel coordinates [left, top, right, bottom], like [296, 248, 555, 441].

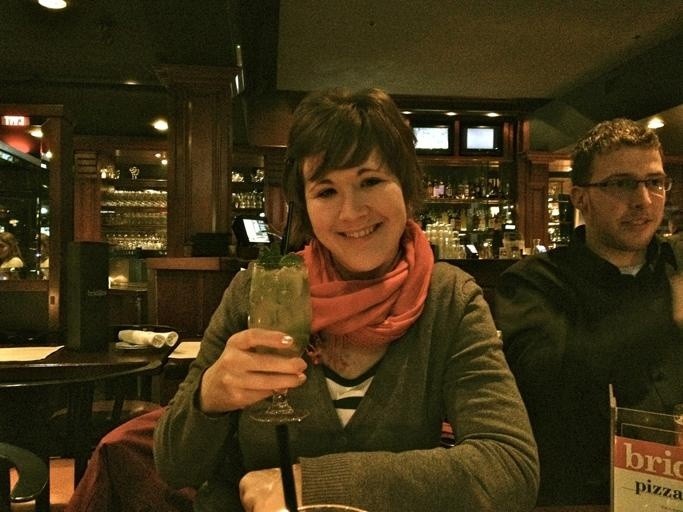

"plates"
[114, 341, 155, 353]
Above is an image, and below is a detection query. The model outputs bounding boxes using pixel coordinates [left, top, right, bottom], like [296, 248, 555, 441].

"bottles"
[422, 174, 541, 259]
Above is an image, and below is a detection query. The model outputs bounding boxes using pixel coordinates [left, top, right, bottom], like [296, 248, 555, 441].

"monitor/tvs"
[231, 215, 271, 247]
[460, 125, 504, 156]
[409, 123, 454, 155]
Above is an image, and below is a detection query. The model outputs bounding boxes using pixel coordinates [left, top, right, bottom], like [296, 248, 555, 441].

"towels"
[117, 326, 177, 348]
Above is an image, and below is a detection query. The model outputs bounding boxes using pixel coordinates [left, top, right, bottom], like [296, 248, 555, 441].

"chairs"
[48, 324, 183, 457]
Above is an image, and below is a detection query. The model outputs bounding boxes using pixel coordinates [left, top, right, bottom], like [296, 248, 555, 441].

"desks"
[0, 326, 163, 512]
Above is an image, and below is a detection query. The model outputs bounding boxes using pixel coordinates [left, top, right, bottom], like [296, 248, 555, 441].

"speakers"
[65, 241, 109, 351]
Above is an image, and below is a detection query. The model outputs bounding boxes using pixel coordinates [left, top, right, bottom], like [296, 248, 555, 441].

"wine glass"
[243, 259, 312, 424]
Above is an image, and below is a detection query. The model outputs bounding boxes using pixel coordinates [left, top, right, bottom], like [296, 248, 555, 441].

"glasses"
[583, 175, 673, 192]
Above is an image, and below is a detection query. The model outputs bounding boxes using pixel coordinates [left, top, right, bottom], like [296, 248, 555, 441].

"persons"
[494, 118, 683, 511]
[153, 83, 541, 511]
[40, 233, 49, 268]
[0, 231, 24, 269]
